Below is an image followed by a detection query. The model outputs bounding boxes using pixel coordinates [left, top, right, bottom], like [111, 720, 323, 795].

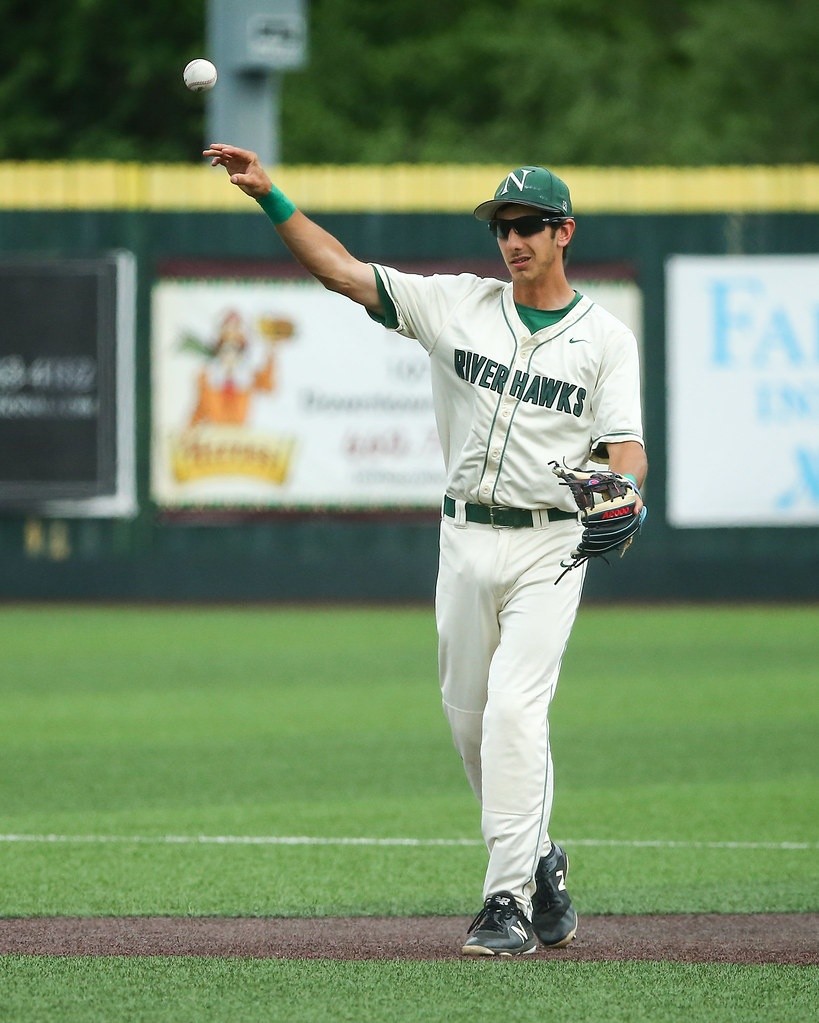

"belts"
[444, 496, 578, 527]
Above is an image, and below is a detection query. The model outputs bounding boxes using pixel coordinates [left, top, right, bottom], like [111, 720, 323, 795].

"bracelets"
[256, 183, 297, 228]
[623, 474, 638, 484]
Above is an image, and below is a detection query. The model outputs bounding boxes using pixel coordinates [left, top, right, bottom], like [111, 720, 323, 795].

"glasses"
[488, 215, 563, 240]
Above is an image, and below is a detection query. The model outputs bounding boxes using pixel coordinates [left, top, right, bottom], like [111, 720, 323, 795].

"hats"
[474, 165, 572, 221]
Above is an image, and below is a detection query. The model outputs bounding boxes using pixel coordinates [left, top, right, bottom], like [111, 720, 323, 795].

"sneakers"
[532, 840, 578, 946]
[462, 891, 538, 956]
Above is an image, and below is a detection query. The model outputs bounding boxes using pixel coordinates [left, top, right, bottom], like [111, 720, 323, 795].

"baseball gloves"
[552, 466, 648, 559]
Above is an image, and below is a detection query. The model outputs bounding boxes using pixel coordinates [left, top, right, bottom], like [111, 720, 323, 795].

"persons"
[202, 142, 648, 956]
[185, 308, 279, 426]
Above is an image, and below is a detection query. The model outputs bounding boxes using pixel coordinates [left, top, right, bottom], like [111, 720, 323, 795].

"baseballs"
[183, 59, 217, 93]
